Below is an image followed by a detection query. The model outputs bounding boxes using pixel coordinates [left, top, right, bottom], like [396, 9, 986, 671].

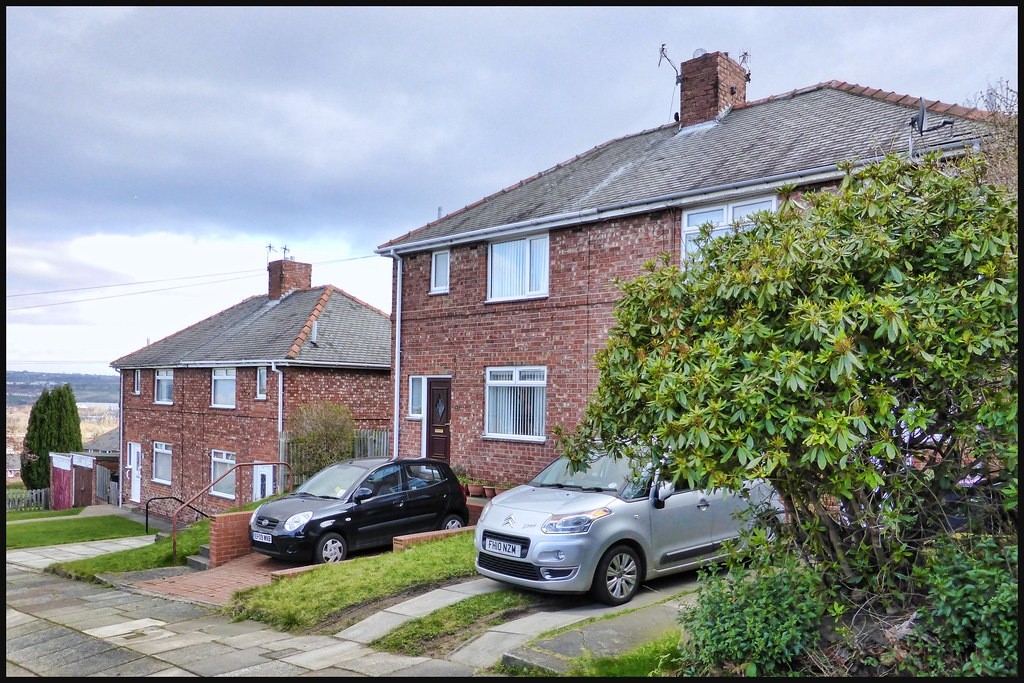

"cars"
[473, 436, 792, 606]
[248, 457, 469, 565]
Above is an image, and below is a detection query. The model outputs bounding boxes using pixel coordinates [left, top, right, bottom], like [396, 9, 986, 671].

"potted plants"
[457, 475, 467, 494]
[468, 479, 490, 497]
[483, 480, 495, 498]
[495, 481, 512, 496]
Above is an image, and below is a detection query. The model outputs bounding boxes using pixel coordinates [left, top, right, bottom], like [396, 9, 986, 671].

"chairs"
[377, 467, 396, 495]
